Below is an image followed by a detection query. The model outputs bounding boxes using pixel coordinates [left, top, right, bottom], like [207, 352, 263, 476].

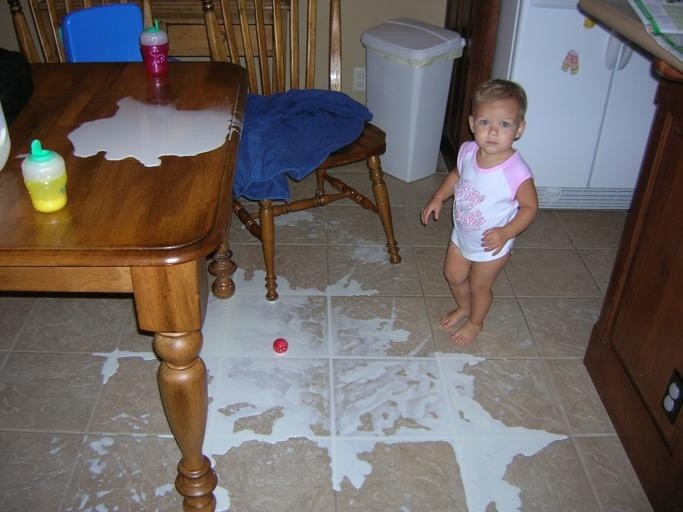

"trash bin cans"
[361, 16, 467, 185]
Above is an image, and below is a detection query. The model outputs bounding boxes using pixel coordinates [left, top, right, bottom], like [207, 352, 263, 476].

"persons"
[421, 79, 538, 345]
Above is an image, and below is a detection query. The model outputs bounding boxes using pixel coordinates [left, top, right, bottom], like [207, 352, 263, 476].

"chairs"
[202, 1, 402, 302]
[8, 0, 153, 63]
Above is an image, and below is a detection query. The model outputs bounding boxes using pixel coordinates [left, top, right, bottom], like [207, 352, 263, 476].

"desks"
[0, 60, 250, 512]
[583, 56, 683, 512]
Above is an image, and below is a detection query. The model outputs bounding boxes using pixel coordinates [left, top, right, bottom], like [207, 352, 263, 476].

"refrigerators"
[487, 0, 663, 212]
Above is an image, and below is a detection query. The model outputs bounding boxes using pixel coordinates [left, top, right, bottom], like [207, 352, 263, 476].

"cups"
[20, 138, 68, 214]
[139, 18, 170, 77]
